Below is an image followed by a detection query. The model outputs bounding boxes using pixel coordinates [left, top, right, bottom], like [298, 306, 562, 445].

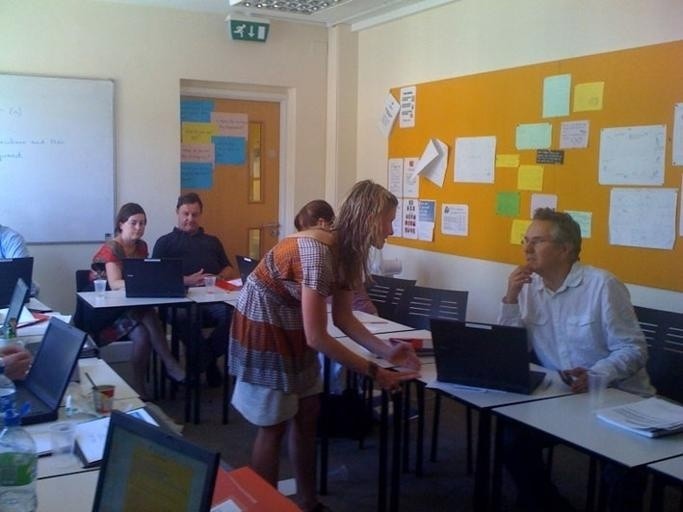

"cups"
[48, 421, 76, 462]
[204, 276, 215, 295]
[93, 280, 106, 299]
[586, 370, 609, 409]
[91, 385, 114, 412]
[0, 337, 23, 349]
[327, 464, 349, 483]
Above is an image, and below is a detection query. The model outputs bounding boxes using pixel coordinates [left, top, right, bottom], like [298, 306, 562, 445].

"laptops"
[1, 278, 27, 340]
[0, 256, 34, 308]
[429, 317, 546, 395]
[236, 253, 260, 286]
[122, 257, 189, 298]
[91, 409, 220, 512]
[3, 316, 87, 426]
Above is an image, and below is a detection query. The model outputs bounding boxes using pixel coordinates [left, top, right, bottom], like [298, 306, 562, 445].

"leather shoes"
[165, 365, 186, 382]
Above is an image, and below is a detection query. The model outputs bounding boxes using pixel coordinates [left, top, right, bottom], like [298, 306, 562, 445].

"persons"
[229, 179, 423, 510]
[0, 344, 30, 385]
[290, 198, 381, 394]
[0, 224, 41, 297]
[150, 190, 234, 388]
[495, 207, 659, 511]
[88, 201, 199, 405]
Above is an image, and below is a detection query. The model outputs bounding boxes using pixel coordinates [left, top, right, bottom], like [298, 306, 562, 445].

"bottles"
[0, 360, 16, 433]
[0, 402, 36, 510]
[369, 247, 385, 277]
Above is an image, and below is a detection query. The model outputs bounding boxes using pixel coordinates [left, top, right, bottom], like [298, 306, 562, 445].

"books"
[594, 392, 683, 442]
[70, 404, 176, 469]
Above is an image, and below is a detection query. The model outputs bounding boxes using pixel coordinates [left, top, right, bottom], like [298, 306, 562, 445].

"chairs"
[360, 275, 418, 325]
[74, 269, 161, 404]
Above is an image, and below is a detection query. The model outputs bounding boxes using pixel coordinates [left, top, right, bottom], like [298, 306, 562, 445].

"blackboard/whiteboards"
[1, 73, 115, 243]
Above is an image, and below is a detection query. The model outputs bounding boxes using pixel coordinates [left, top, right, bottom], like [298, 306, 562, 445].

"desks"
[0, 307, 181, 511]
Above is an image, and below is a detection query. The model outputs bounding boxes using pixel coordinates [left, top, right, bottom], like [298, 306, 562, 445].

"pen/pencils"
[85, 372, 97, 389]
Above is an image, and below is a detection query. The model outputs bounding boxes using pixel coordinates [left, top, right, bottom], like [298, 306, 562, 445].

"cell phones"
[557, 368, 573, 389]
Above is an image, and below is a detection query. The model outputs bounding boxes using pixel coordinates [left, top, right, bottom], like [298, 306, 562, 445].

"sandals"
[306, 502, 332, 512]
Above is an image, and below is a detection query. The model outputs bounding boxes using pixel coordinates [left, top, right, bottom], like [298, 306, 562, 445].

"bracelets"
[365, 361, 380, 381]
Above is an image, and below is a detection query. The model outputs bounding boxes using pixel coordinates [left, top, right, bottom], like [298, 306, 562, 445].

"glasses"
[521, 238, 552, 246]
